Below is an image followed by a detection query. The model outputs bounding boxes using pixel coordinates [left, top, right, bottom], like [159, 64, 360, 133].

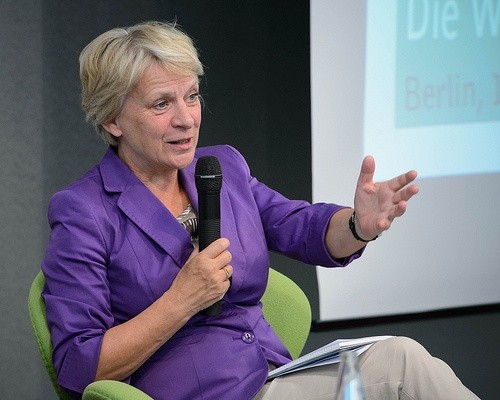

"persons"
[41, 22, 478, 400]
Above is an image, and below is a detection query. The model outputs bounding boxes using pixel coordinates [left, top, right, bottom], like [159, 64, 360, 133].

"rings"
[222, 267, 231, 280]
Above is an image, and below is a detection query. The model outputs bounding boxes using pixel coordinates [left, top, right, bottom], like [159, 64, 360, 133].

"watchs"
[349, 211, 381, 242]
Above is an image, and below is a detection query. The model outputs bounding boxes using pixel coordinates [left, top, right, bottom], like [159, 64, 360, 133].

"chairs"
[28, 266, 311, 400]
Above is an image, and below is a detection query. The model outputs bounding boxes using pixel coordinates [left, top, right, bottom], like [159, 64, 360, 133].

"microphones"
[194, 156, 223, 316]
